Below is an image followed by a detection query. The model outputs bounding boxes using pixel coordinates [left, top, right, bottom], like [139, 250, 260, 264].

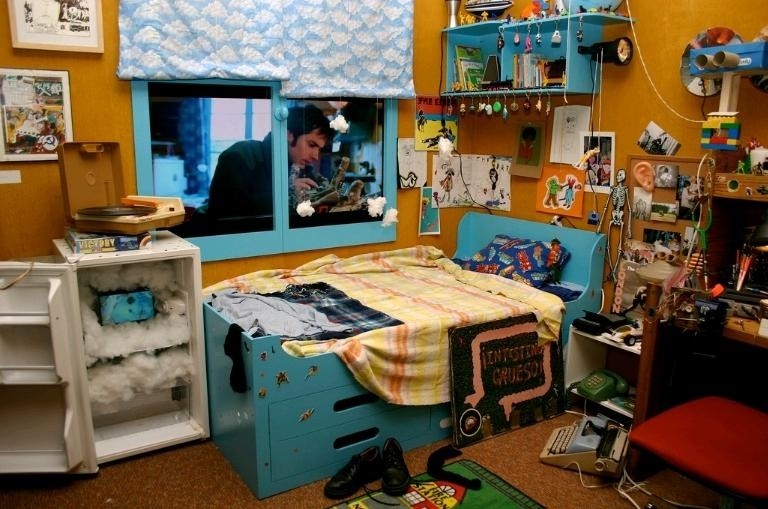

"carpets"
[324, 459, 550, 508]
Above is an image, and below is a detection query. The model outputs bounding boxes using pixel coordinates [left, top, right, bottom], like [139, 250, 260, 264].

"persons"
[681, 180, 698, 214]
[585, 144, 610, 187]
[208, 105, 335, 218]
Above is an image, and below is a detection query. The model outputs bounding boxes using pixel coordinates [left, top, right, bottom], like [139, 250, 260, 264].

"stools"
[625, 392, 768, 509]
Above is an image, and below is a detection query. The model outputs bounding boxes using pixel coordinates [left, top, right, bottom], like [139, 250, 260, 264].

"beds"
[200, 212, 608, 502]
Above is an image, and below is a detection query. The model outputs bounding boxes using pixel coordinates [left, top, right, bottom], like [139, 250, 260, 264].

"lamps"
[578, 36, 634, 66]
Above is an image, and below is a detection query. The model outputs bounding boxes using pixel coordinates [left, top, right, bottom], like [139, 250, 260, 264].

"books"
[514, 53, 566, 90]
[453, 45, 485, 92]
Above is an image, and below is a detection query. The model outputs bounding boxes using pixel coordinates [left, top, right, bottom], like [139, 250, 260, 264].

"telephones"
[577, 368, 630, 401]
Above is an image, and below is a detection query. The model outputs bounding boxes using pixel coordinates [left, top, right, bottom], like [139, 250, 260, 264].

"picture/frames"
[0, 1, 105, 163]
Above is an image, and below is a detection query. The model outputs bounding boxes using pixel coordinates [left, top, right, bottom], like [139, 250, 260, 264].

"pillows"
[461, 235, 572, 289]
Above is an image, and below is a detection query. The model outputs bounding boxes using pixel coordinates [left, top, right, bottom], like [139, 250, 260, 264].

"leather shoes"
[324, 437, 411, 499]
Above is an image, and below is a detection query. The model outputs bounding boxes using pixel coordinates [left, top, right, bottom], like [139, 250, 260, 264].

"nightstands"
[562, 312, 642, 418]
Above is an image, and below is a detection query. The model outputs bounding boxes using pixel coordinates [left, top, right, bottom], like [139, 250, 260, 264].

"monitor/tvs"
[704, 193, 767, 298]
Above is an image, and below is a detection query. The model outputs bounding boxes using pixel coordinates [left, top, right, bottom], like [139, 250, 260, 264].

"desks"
[626, 165, 768, 478]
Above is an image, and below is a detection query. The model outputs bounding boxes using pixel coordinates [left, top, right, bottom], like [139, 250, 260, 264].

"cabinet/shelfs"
[0, 229, 211, 474]
[440, 0, 637, 95]
